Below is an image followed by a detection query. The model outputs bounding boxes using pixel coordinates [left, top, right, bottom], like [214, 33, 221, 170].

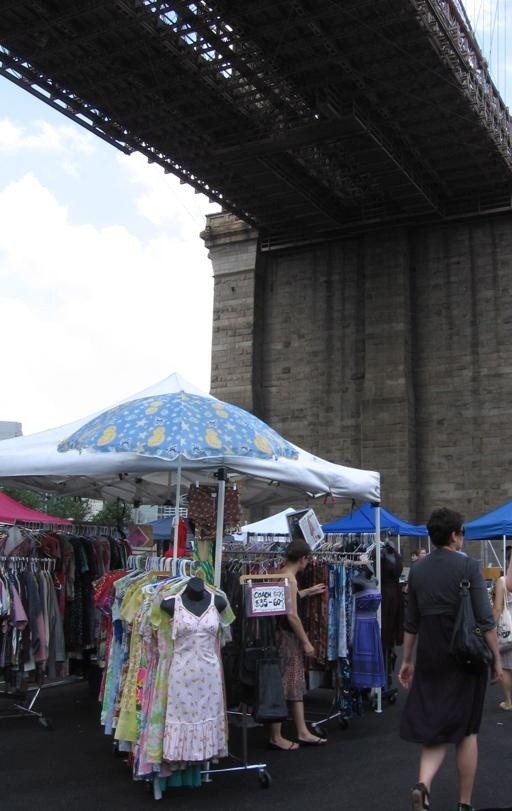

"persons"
[266, 541, 327, 752]
[495, 543, 511, 711]
[353, 568, 383, 691]
[408, 551, 417, 562]
[397, 507, 503, 811]
[377, 542, 404, 662]
[417, 547, 426, 557]
[162, 578, 228, 771]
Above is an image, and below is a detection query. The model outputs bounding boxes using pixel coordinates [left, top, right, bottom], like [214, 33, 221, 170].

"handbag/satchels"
[449, 586, 494, 666]
[495, 607, 512, 654]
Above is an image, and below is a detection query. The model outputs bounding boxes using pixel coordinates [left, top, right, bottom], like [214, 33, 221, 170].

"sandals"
[454, 801, 475, 811]
[296, 736, 329, 745]
[407, 783, 432, 811]
[266, 739, 300, 753]
[500, 699, 512, 711]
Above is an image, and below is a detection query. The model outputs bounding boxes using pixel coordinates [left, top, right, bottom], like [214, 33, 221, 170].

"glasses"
[454, 527, 467, 536]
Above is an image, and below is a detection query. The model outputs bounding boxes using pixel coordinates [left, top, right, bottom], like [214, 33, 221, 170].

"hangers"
[124, 553, 232, 609]
[3, 519, 123, 553]
[235, 530, 384, 568]
[1, 556, 63, 590]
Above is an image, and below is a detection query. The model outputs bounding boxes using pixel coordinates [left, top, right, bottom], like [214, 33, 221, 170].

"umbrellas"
[56, 389, 300, 559]
